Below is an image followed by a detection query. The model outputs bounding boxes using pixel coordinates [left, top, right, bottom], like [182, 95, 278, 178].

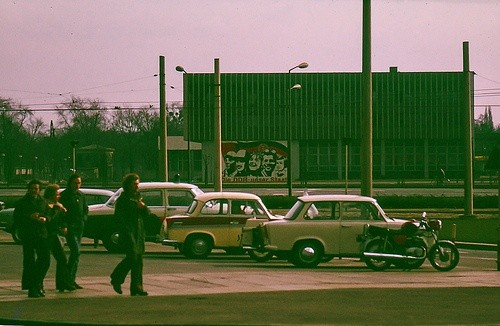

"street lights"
[32, 155, 39, 178]
[288, 62, 310, 197]
[175, 65, 191, 184]
[2, 152, 6, 183]
[287, 84, 301, 182]
[18, 153, 24, 183]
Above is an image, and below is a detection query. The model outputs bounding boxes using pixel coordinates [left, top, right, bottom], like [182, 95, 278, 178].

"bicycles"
[430, 173, 450, 188]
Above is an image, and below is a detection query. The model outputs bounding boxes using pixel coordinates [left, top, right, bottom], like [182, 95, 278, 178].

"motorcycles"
[355, 212, 460, 273]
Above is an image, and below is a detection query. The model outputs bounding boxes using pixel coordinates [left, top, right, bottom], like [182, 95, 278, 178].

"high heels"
[130, 290, 148, 296]
[110, 281, 122, 294]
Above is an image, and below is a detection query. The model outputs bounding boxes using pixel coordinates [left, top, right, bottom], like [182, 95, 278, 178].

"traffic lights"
[70, 141, 79, 148]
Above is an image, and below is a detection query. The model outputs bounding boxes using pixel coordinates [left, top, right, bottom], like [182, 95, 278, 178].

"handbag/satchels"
[34, 223, 53, 249]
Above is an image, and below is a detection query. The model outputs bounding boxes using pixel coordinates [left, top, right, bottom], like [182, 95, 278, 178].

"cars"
[239, 194, 438, 269]
[82, 181, 264, 254]
[0, 188, 116, 244]
[160, 191, 319, 263]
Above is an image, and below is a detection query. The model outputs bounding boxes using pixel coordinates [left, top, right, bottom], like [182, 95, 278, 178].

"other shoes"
[74, 283, 83, 289]
[27, 289, 45, 297]
[59, 286, 75, 293]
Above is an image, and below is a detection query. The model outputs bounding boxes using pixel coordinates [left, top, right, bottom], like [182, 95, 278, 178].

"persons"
[13, 174, 88, 297]
[438, 168, 445, 182]
[110, 173, 150, 296]
